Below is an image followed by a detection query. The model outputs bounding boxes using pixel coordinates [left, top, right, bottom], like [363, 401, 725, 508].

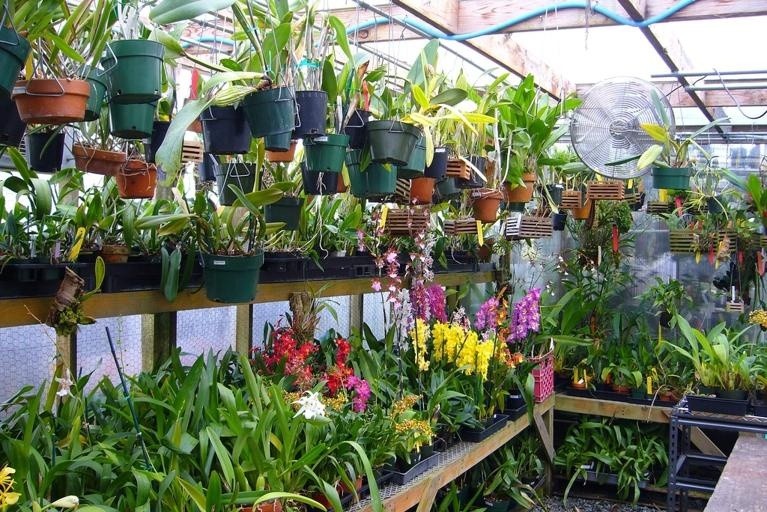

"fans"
[569, 73, 677, 181]
